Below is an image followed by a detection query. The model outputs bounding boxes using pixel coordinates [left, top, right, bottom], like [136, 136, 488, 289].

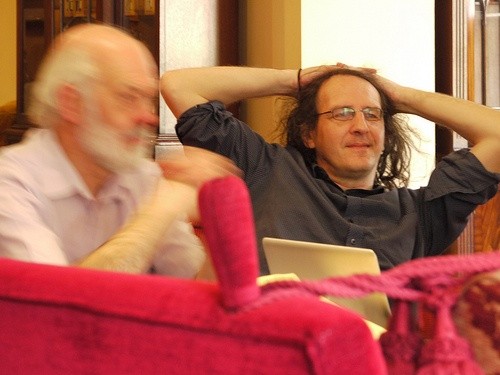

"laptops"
[262, 238, 391, 330]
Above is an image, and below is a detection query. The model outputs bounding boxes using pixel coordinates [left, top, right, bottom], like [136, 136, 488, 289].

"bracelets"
[297, 68, 303, 94]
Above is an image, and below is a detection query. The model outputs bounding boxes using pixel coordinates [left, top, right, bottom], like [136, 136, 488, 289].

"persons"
[162, 56, 500, 279]
[0, 25, 243, 283]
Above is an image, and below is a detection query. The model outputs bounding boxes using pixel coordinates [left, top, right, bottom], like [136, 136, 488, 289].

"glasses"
[315, 106, 383, 120]
